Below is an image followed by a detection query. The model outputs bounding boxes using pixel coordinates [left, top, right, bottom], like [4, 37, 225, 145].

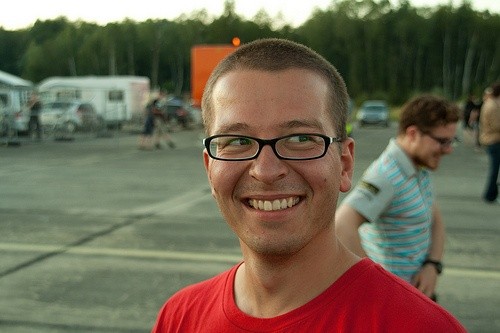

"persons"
[465, 84, 500, 202]
[151, 37, 467, 333]
[139, 89, 193, 152]
[24, 90, 45, 146]
[334, 93, 461, 303]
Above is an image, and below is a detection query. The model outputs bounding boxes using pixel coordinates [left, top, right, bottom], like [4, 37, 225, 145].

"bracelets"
[422, 258, 443, 272]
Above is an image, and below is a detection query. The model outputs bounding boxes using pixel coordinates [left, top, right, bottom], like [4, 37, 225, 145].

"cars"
[155, 98, 190, 128]
[356, 100, 390, 129]
[14, 100, 107, 137]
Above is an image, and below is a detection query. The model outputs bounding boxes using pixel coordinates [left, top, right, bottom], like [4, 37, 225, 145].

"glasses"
[423, 131, 459, 146]
[202, 133, 344, 161]
